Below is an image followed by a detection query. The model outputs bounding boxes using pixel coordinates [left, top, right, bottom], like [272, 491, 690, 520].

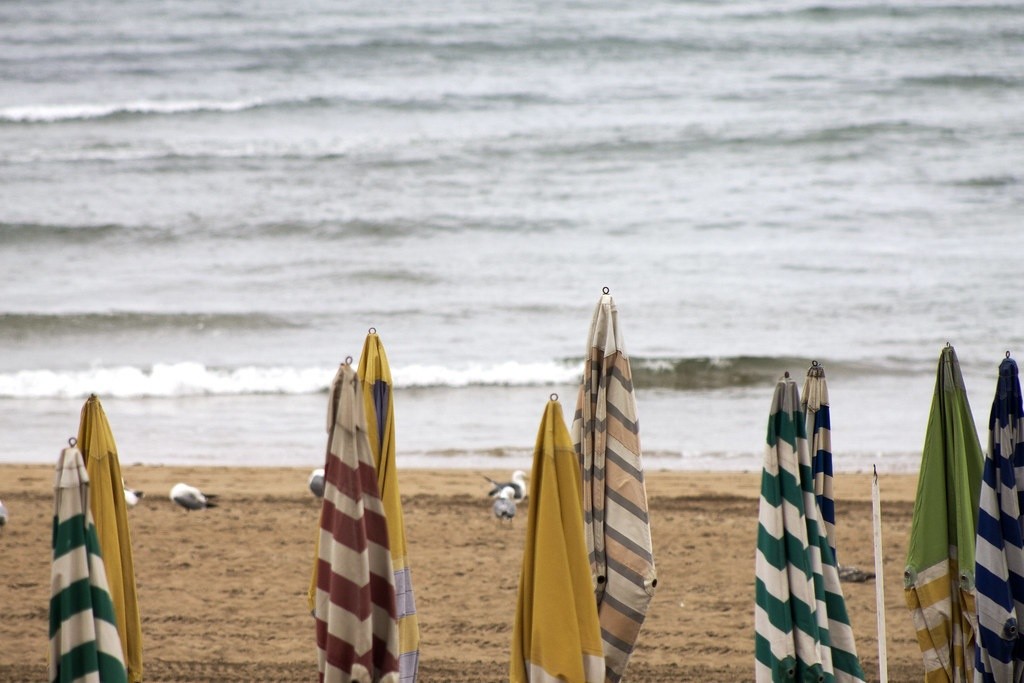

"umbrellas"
[306, 328, 420, 683]
[974, 350, 1024, 683]
[755, 360, 866, 683]
[509, 287, 657, 683]
[904, 342, 986, 683]
[46, 393, 144, 683]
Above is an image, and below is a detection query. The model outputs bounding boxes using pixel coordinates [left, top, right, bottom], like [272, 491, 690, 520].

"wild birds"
[477, 468, 531, 530]
[306, 468, 324, 502]
[121, 476, 220, 513]
